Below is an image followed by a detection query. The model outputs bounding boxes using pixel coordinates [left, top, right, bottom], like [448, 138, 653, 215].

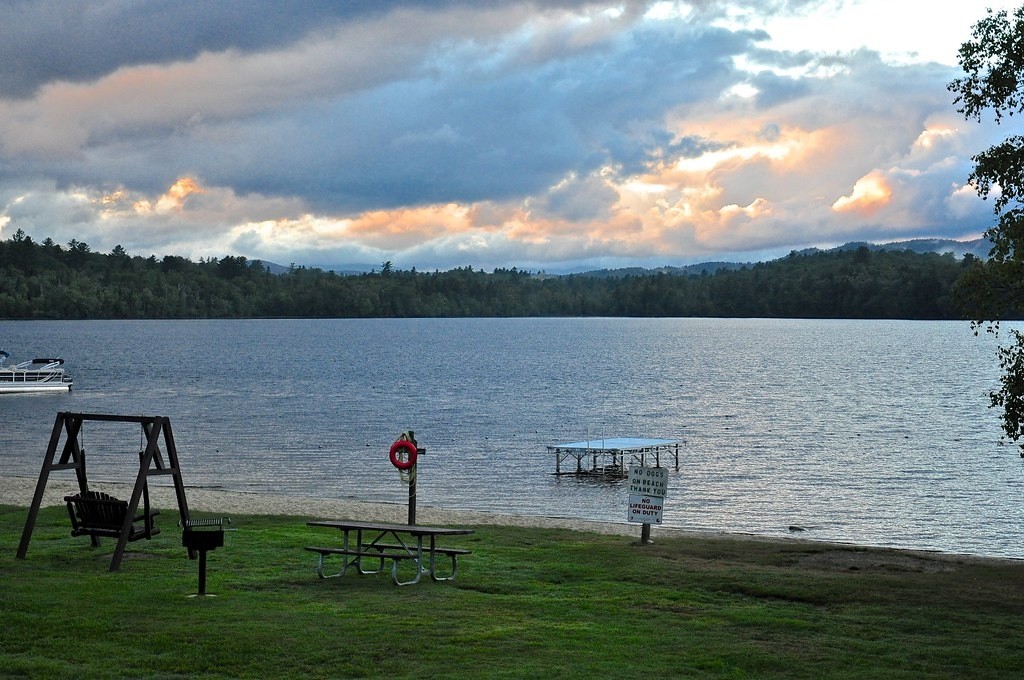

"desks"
[308, 521, 475, 586]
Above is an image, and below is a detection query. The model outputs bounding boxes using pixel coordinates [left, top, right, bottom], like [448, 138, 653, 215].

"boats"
[0, 351, 74, 394]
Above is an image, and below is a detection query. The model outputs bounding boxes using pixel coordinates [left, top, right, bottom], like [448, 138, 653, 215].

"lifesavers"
[389, 441, 417, 469]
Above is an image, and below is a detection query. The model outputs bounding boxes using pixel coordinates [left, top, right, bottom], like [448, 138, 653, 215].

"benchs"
[305, 543, 472, 586]
